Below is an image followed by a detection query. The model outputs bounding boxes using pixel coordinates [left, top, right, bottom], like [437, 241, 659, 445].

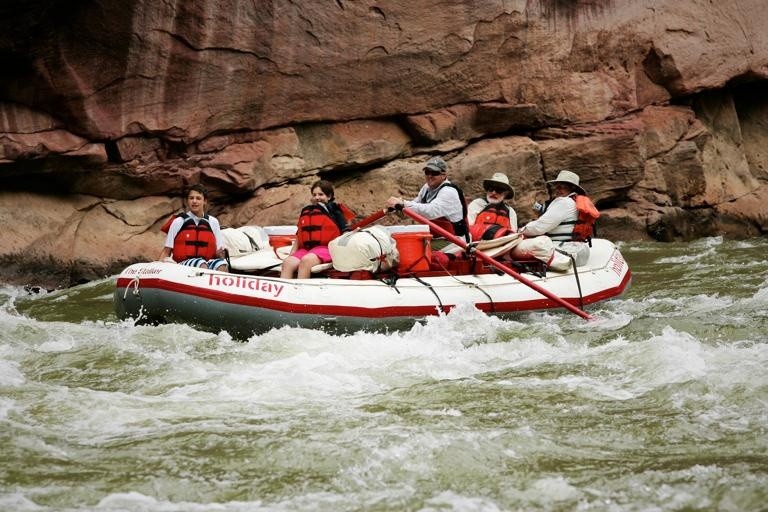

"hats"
[422, 157, 449, 173]
[483, 173, 515, 200]
[546, 170, 587, 195]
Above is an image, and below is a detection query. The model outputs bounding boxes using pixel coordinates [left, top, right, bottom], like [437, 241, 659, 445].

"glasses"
[424, 167, 443, 176]
[552, 182, 576, 191]
[488, 184, 508, 194]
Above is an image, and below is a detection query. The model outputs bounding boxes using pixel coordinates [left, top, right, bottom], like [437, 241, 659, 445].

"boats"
[114, 219, 632, 343]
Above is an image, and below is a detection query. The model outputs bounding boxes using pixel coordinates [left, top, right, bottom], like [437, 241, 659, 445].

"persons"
[278, 178, 358, 278]
[518, 168, 600, 241]
[157, 183, 229, 273]
[385, 154, 468, 251]
[467, 171, 518, 242]
